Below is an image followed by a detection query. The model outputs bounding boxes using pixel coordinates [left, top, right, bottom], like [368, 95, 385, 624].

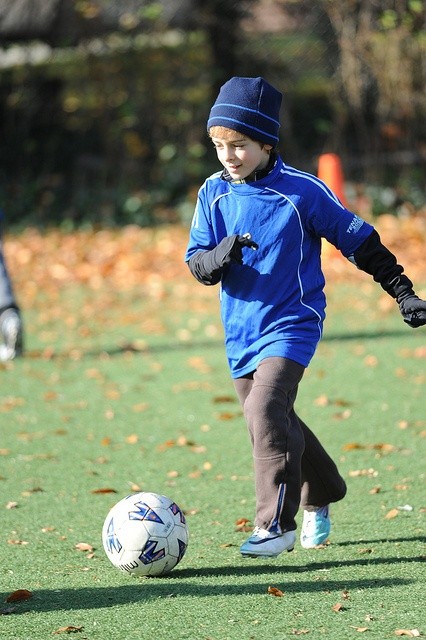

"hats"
[205, 75, 283, 151]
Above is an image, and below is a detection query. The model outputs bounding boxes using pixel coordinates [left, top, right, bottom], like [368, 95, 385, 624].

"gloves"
[395, 287, 426, 328]
[186, 234, 259, 286]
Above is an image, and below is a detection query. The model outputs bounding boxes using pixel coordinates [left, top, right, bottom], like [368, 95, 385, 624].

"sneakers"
[238, 526, 298, 559]
[300, 504, 331, 549]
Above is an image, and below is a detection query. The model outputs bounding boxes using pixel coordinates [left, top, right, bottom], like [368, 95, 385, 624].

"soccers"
[102, 492, 189, 578]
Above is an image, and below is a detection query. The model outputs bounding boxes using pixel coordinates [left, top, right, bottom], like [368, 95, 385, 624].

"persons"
[186, 76, 425, 558]
[1, 253, 24, 364]
